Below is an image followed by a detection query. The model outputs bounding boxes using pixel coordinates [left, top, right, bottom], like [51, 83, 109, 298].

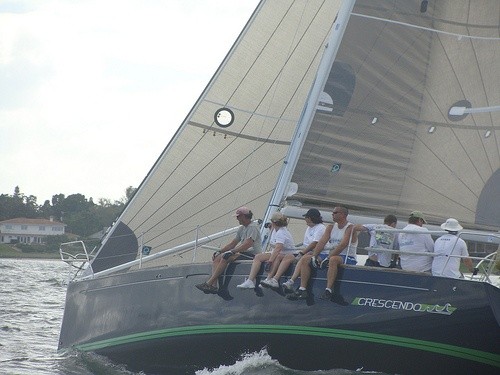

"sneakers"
[236, 277, 256, 289]
[283, 281, 293, 290]
[321, 289, 334, 298]
[260, 279, 279, 288]
[287, 288, 309, 300]
[195, 282, 217, 294]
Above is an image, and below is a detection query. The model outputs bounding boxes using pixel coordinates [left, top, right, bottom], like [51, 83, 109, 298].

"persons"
[432, 218, 474, 279]
[287, 204, 359, 300]
[359, 214, 397, 268]
[260, 209, 330, 299]
[397, 209, 434, 272]
[236, 211, 295, 289]
[195, 207, 263, 296]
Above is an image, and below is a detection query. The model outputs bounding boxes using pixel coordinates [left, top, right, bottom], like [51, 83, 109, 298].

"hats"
[232, 207, 251, 217]
[302, 209, 320, 218]
[408, 211, 426, 223]
[270, 212, 283, 222]
[441, 218, 463, 231]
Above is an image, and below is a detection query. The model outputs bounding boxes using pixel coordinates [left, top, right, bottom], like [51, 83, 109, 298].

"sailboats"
[57, 0, 500, 375]
